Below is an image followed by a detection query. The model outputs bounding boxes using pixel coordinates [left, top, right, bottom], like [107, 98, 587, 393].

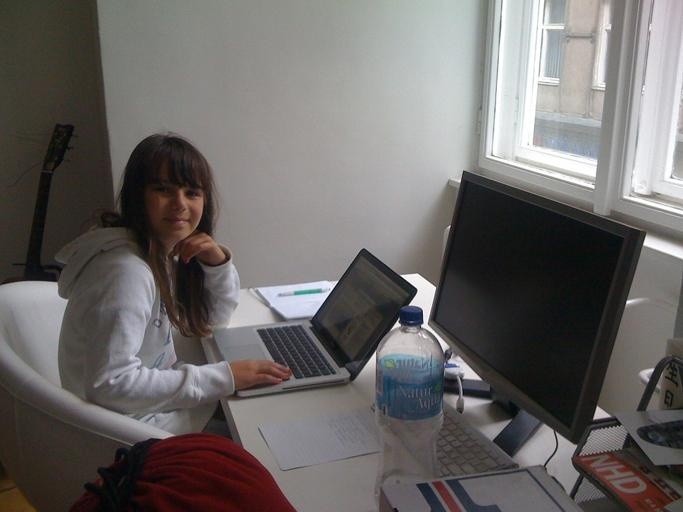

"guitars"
[1, 123, 78, 282]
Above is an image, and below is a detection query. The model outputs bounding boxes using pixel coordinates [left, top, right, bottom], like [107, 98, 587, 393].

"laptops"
[211, 246, 417, 396]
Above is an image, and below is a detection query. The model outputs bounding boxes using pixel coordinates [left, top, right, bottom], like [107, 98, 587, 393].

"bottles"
[374, 305, 443, 505]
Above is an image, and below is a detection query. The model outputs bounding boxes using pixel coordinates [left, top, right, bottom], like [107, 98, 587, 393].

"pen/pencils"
[278, 288, 330, 296]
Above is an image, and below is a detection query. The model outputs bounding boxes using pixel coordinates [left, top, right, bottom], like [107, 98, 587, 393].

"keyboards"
[369, 396, 520, 480]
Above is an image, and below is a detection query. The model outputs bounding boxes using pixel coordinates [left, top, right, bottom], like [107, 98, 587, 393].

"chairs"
[1, 279, 205, 512]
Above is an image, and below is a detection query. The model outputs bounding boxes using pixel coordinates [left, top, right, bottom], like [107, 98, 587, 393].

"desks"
[200, 273, 624, 509]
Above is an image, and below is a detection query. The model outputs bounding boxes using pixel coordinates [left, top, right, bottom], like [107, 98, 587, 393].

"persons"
[53, 134, 292, 439]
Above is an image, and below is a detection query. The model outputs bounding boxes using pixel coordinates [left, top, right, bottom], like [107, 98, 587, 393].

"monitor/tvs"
[427, 166, 647, 459]
